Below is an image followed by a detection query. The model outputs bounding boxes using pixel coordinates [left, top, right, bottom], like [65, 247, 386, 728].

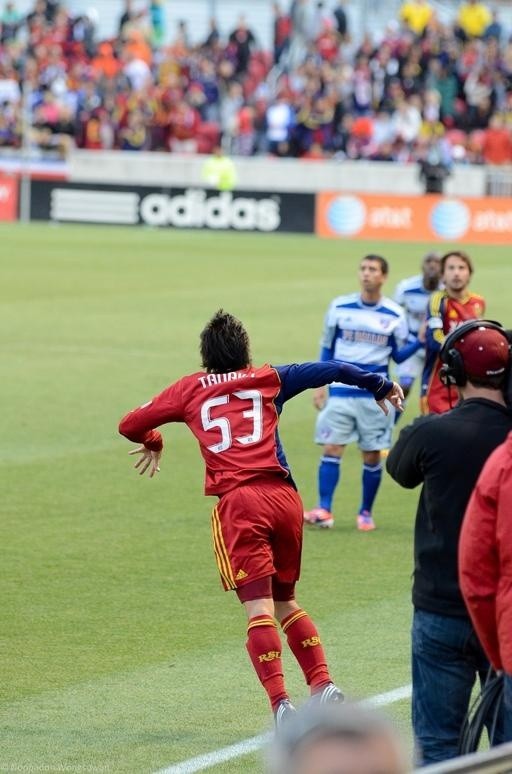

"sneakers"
[273, 698, 297, 743]
[304, 680, 345, 721]
[357, 509, 376, 532]
[303, 507, 335, 529]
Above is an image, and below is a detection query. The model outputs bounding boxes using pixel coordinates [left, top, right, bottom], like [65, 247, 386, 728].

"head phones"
[438, 319, 512, 387]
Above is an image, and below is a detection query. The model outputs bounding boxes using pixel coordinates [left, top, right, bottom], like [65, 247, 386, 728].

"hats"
[453, 326, 511, 377]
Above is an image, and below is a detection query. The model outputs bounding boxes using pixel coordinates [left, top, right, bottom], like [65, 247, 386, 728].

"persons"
[118, 308, 406, 738]
[419, 250, 487, 417]
[390, 253, 446, 427]
[456, 431, 512, 743]
[301, 253, 429, 532]
[384, 319, 512, 770]
